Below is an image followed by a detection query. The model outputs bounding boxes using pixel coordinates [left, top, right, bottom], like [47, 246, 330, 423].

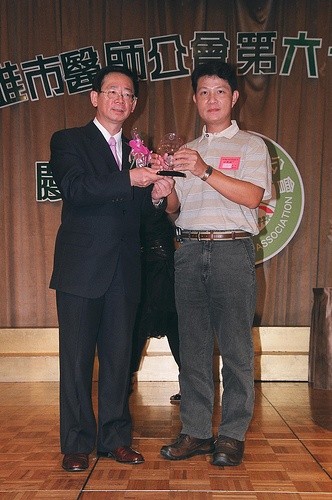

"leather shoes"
[62, 453, 88, 473]
[98, 446, 144, 463]
[160, 434, 215, 460]
[213, 433, 244, 465]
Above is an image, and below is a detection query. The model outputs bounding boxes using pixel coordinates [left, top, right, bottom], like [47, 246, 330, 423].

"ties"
[108, 137, 120, 170]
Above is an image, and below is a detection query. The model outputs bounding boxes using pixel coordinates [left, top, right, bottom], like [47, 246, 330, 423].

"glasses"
[96, 91, 138, 104]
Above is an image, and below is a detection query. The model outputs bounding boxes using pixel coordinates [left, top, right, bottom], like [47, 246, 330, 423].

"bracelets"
[201, 165, 213, 181]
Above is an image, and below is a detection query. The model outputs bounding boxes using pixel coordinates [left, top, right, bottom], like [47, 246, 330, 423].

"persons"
[49, 65, 175, 470]
[148, 62, 273, 466]
[125, 208, 182, 402]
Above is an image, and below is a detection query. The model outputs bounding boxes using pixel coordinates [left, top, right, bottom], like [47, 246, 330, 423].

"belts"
[180, 231, 250, 241]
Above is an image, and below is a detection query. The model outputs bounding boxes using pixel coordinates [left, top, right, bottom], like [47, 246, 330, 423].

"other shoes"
[170, 393, 180, 400]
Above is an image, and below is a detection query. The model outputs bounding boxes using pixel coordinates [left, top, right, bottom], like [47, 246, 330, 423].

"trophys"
[156, 132, 188, 177]
